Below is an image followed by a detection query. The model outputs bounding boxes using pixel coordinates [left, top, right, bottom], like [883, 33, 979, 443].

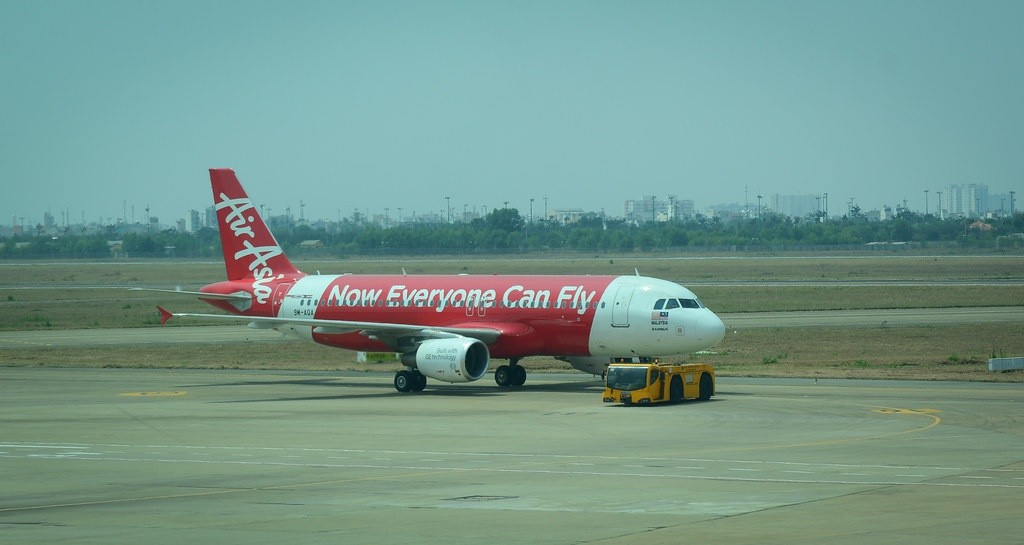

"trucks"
[600, 362, 717, 406]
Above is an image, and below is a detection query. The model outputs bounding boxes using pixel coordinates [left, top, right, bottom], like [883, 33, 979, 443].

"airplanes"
[125, 165, 729, 395]
